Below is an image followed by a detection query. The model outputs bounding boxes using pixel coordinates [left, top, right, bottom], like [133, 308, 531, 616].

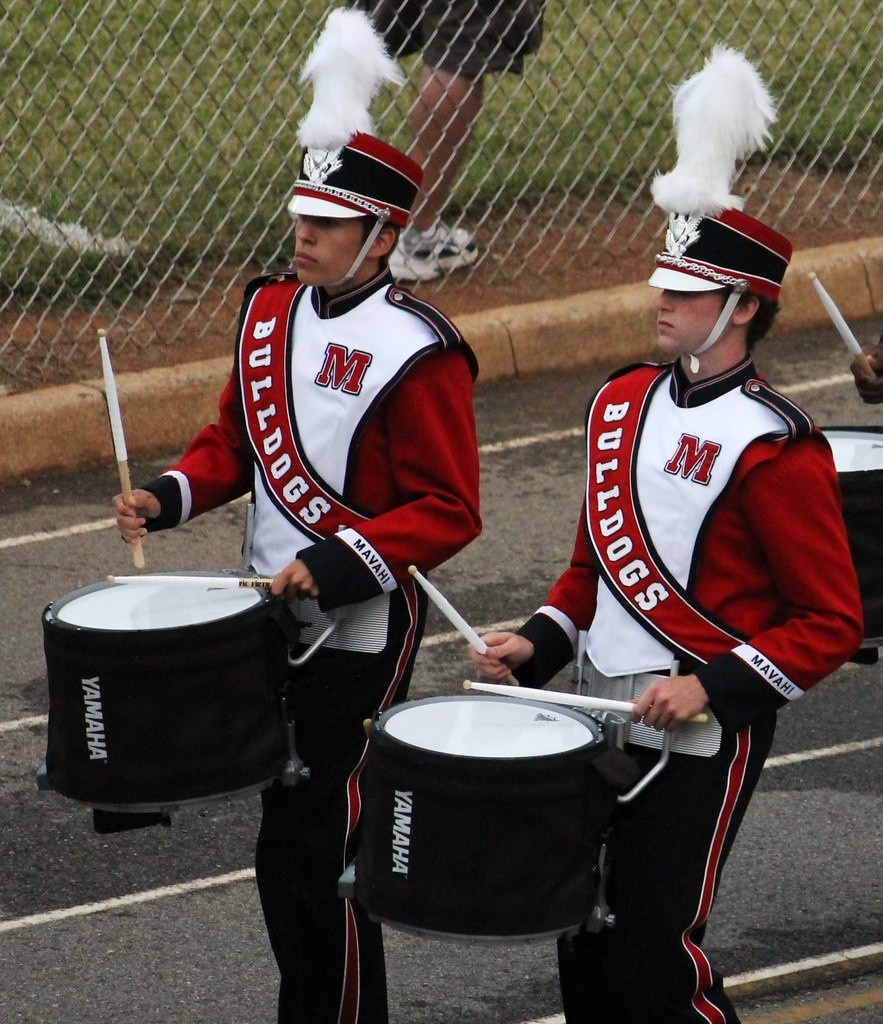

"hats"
[649, 205, 792, 296]
[286, 134, 424, 230]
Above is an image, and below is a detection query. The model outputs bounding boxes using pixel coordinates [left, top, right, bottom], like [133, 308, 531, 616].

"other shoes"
[388, 229, 479, 280]
[847, 647, 879, 665]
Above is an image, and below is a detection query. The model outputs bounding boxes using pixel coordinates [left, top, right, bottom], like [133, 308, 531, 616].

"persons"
[113, 9, 482, 1023]
[466, 43, 863, 1024]
[851, 338, 881, 403]
[342, 1, 545, 280]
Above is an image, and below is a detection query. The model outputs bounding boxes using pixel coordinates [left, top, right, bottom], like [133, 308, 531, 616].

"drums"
[34, 566, 292, 818]
[350, 690, 618, 944]
[817, 423, 883, 641]
